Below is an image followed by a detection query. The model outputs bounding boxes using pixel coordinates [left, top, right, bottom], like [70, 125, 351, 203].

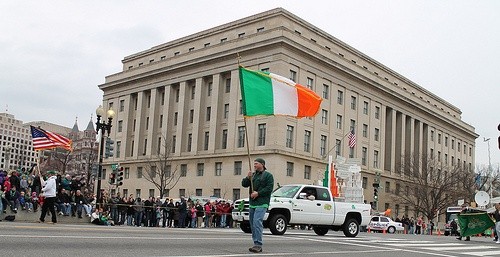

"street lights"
[96, 104, 115, 208]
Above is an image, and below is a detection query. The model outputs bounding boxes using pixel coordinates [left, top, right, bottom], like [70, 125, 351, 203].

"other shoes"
[249, 246, 262, 253]
[34, 220, 42, 223]
[464, 239, 470, 241]
[456, 237, 461, 241]
[47, 221, 55, 224]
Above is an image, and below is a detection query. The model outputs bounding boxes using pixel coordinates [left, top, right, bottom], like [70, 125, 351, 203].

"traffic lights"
[109, 173, 115, 184]
[373, 183, 380, 187]
[374, 189, 378, 200]
[115, 164, 123, 186]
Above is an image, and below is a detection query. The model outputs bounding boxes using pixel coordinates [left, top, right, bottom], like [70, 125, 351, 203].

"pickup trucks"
[231, 184, 371, 237]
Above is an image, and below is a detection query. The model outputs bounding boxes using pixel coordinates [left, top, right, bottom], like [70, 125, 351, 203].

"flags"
[30, 125, 71, 150]
[238, 66, 323, 118]
[458, 212, 496, 236]
[348, 129, 356, 148]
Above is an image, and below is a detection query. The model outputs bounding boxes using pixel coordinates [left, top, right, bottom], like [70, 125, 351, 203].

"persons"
[242, 158, 274, 252]
[456, 203, 500, 243]
[0, 166, 234, 228]
[395, 214, 434, 234]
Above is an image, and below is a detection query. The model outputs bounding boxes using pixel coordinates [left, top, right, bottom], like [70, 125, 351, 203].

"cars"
[366, 215, 404, 233]
[444, 219, 460, 236]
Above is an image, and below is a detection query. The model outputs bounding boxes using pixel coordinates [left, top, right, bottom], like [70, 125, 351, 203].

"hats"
[47, 170, 55, 175]
[254, 157, 266, 170]
